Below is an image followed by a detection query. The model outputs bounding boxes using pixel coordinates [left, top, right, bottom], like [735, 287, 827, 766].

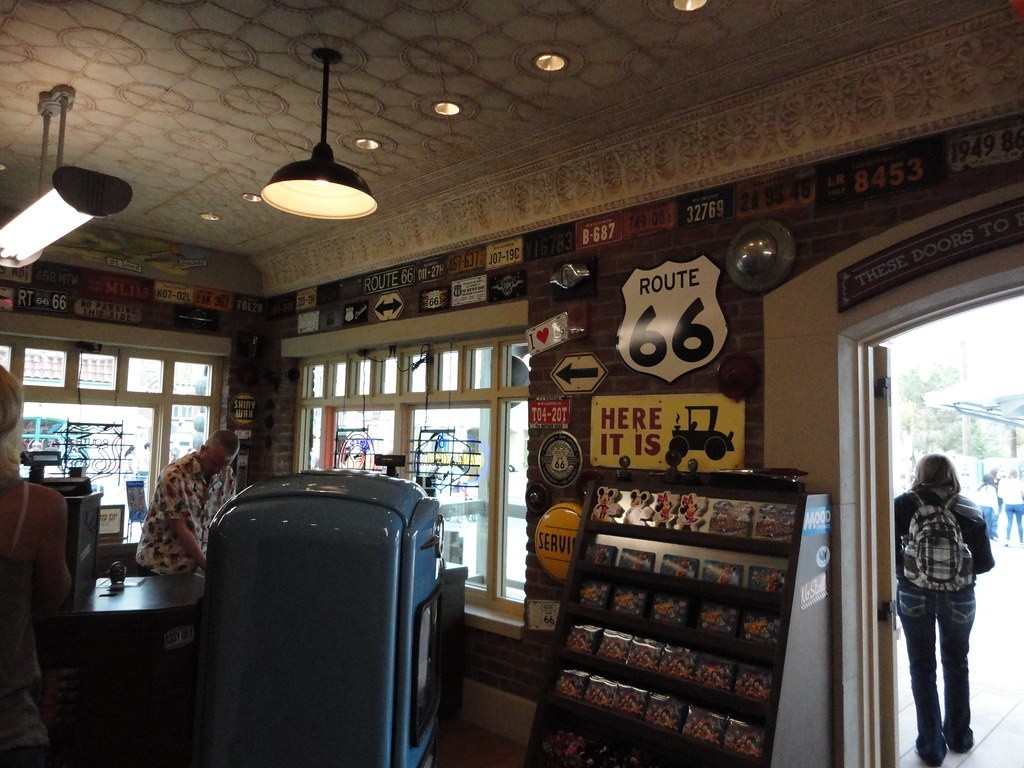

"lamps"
[260, 46, 380, 221]
[0, 82, 135, 271]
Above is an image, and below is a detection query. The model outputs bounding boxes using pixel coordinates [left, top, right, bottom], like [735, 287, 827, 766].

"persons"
[998, 469, 1024, 547]
[976, 467, 1003, 542]
[894, 455, 996, 767]
[0, 366, 73, 768]
[135, 430, 240, 579]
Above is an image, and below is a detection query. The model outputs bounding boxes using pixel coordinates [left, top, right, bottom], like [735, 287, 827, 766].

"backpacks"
[903, 491, 974, 591]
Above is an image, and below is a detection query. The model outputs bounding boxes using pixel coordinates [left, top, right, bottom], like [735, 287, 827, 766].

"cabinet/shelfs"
[520, 465, 837, 768]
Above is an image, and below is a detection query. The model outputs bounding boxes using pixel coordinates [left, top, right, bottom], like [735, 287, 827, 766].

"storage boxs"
[541, 543, 787, 768]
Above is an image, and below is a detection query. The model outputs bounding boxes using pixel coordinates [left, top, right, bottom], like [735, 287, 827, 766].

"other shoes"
[1021, 542, 1024, 548]
[1004, 540, 1010, 547]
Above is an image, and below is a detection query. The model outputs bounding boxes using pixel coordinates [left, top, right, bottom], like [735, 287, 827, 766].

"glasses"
[206, 448, 229, 469]
